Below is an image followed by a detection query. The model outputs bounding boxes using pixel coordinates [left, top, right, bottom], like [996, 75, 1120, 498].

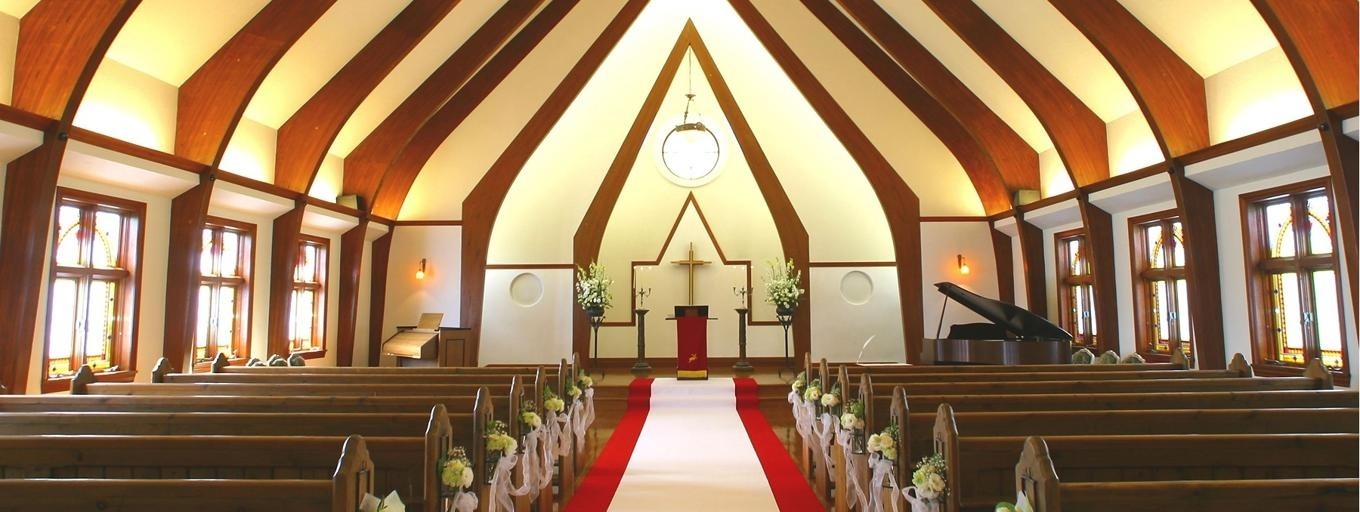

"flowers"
[763, 256, 805, 309]
[788, 369, 1036, 512]
[577, 257, 615, 313]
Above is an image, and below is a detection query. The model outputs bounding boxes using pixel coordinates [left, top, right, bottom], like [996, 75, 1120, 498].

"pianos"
[933, 283, 1073, 365]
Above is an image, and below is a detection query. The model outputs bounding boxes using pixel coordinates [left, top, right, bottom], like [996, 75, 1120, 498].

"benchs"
[152, 358, 574, 508]
[70, 364, 557, 509]
[210, 353, 594, 475]
[836, 367, 1358, 508]
[817, 358, 1333, 504]
[802, 351, 1255, 481]
[2, 386, 499, 510]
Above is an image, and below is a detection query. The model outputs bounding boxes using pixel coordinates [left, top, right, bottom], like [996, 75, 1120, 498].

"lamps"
[958, 255, 969, 274]
[415, 258, 426, 279]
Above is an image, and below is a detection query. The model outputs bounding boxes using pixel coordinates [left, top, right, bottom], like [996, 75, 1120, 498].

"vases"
[587, 307, 605, 316]
[776, 303, 794, 315]
